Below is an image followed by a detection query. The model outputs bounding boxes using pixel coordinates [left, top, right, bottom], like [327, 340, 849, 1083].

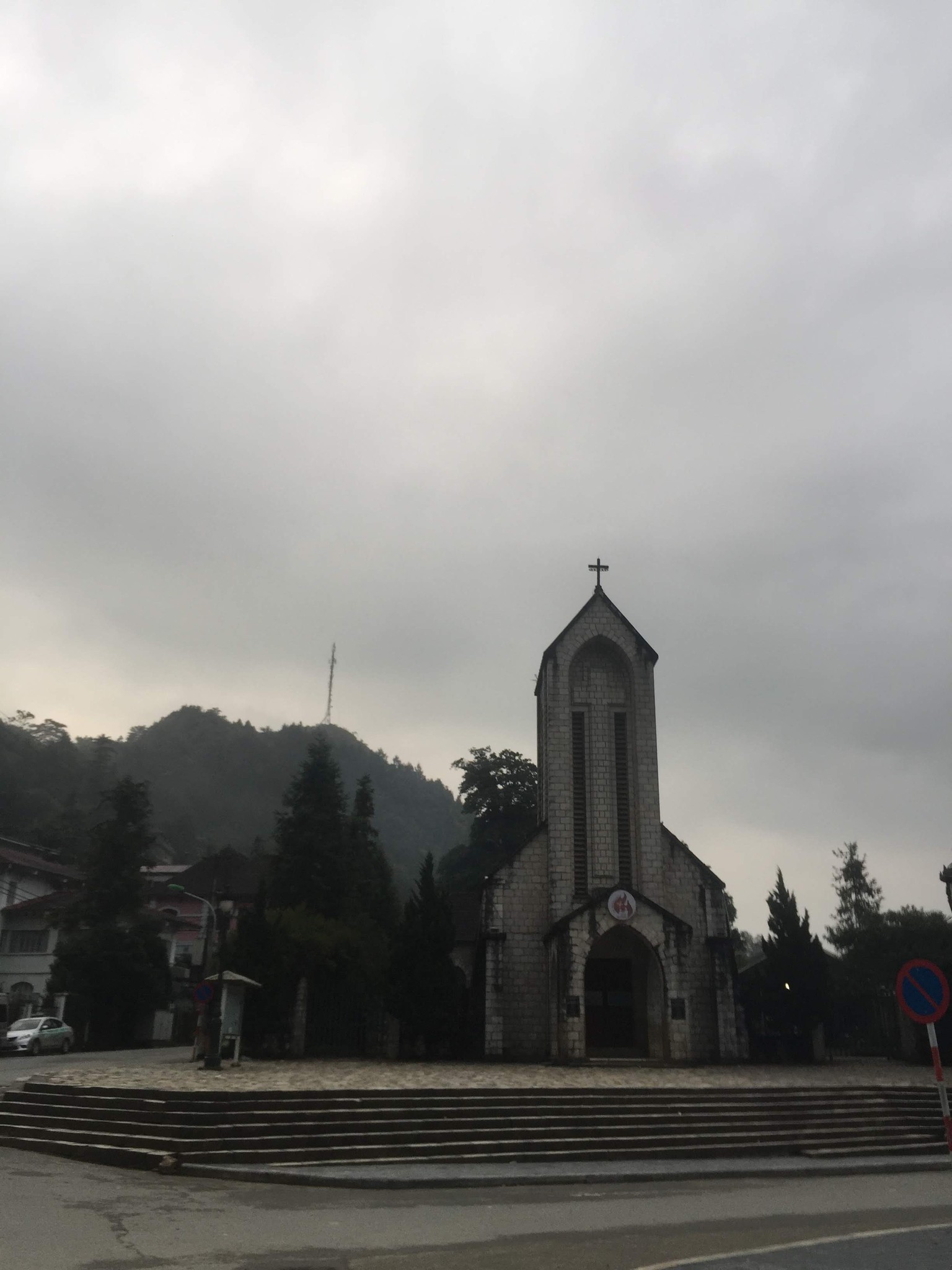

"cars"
[0, 1017, 76, 1055]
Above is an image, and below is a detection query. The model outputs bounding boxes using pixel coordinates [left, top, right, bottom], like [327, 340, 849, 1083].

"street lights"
[167, 883, 224, 1071]
